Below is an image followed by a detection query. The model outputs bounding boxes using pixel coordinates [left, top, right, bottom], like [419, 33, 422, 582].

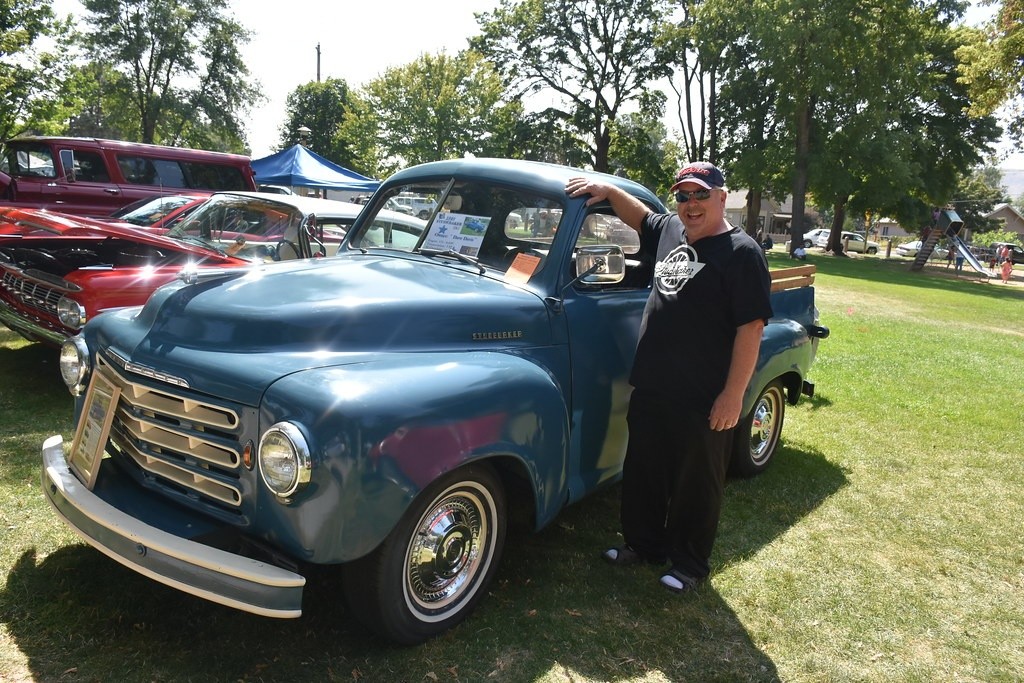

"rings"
[724, 423, 731, 426]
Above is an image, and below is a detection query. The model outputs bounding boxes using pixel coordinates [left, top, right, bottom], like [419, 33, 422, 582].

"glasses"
[674, 189, 721, 202]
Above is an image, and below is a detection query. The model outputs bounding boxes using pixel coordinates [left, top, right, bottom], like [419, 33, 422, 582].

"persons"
[529, 208, 555, 238]
[790, 243, 807, 261]
[563, 161, 773, 592]
[921, 202, 1013, 284]
[752, 227, 774, 251]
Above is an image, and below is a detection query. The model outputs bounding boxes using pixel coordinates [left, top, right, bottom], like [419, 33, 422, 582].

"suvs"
[817, 229, 880, 255]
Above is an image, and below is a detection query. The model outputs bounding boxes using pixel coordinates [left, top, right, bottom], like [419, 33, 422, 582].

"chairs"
[501, 249, 547, 279]
[602, 260, 646, 290]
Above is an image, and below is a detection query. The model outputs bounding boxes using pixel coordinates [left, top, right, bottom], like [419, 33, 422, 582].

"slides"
[946, 227, 997, 278]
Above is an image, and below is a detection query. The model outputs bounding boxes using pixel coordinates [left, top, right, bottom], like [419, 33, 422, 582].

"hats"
[670, 162, 724, 190]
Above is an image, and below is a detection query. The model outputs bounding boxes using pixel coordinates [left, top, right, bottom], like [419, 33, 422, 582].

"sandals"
[602, 543, 650, 565]
[660, 566, 705, 593]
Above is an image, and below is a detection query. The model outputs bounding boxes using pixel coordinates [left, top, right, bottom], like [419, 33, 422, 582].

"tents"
[250, 143, 383, 204]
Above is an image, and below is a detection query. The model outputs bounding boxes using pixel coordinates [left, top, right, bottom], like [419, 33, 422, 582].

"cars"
[802, 229, 824, 248]
[966, 241, 1024, 265]
[529, 208, 641, 246]
[506, 213, 522, 230]
[896, 241, 950, 260]
[0, 138, 438, 345]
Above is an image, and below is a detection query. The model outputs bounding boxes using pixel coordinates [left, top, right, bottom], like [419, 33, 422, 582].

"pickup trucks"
[43, 157, 832, 648]
